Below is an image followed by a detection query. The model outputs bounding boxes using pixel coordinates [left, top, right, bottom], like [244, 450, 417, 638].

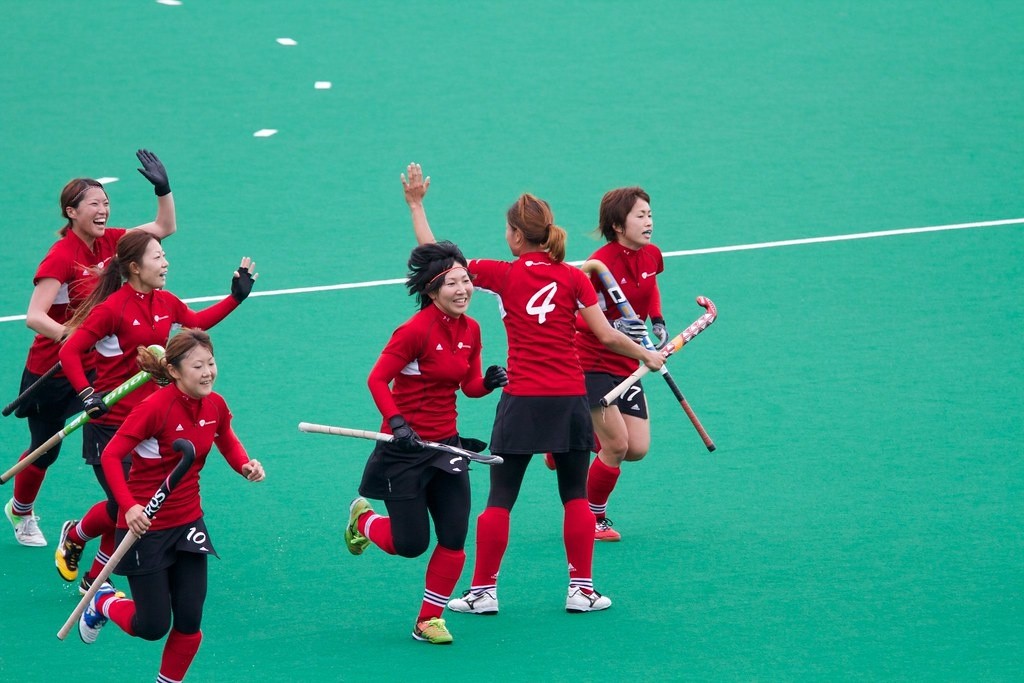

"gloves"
[652, 319, 668, 349]
[137, 149, 171, 196]
[79, 387, 110, 418]
[388, 415, 421, 453]
[609, 315, 648, 342]
[484, 366, 508, 391]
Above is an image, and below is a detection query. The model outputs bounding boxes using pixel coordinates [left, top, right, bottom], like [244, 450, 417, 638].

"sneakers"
[412, 617, 453, 643]
[54, 521, 83, 582]
[78, 583, 115, 644]
[79, 572, 111, 595]
[545, 453, 556, 470]
[5, 498, 47, 547]
[565, 585, 612, 611]
[345, 497, 373, 556]
[594, 517, 620, 541]
[448, 588, 499, 614]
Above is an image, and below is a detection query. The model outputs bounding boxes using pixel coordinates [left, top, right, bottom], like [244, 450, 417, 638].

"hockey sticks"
[0, 343, 167, 486]
[1, 360, 62, 418]
[599, 295, 718, 409]
[298, 419, 505, 466]
[55, 438, 197, 643]
[580, 258, 717, 455]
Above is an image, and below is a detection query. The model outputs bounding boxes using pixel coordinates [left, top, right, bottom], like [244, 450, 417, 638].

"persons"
[543, 187, 669, 542]
[78, 329, 264, 683]
[399, 162, 666, 613]
[55, 229, 259, 597]
[346, 242, 509, 644]
[3, 148, 176, 547]
